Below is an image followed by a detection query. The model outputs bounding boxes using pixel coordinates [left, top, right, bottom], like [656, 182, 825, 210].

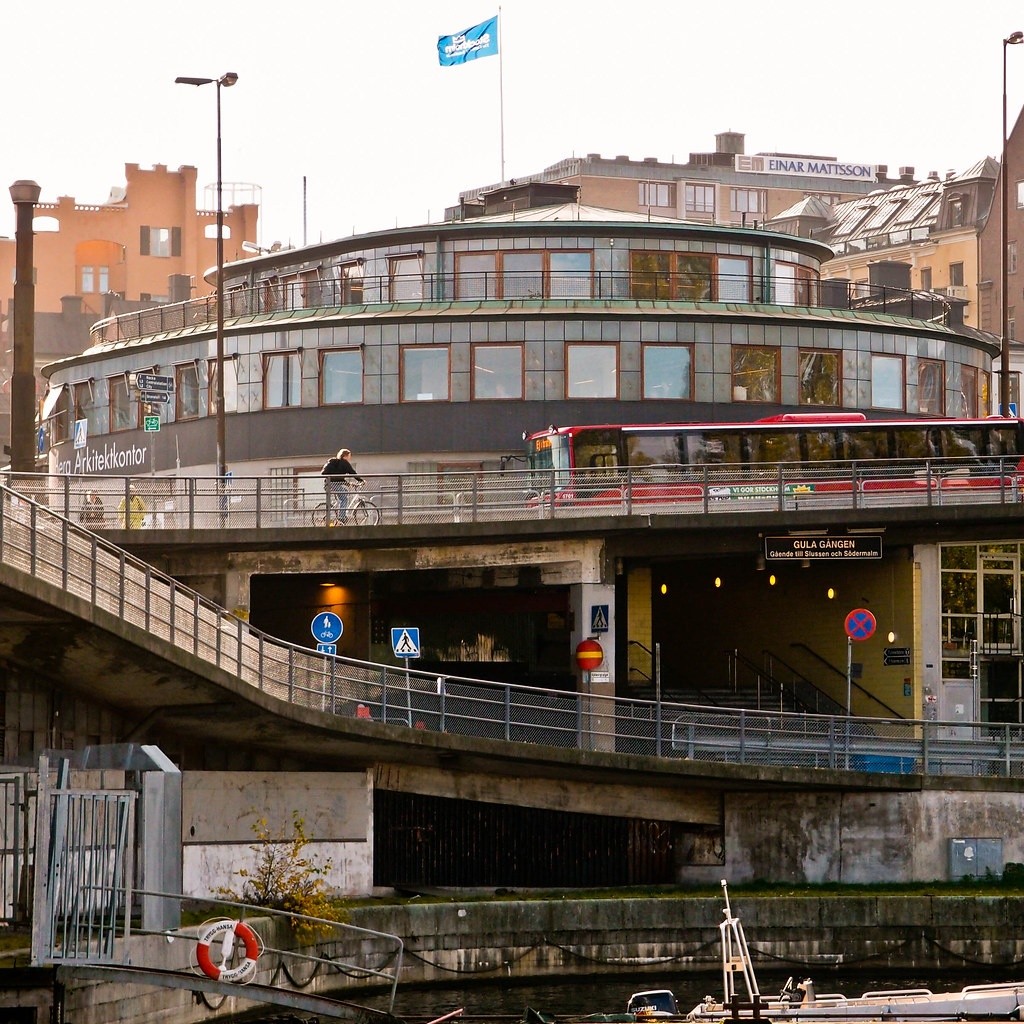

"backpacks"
[320, 457, 340, 474]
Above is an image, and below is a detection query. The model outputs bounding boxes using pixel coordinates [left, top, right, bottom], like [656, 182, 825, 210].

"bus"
[524, 409, 1024, 507]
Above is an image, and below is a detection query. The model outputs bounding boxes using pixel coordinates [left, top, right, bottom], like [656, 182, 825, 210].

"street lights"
[999, 29, 1022, 420]
[177, 71, 239, 530]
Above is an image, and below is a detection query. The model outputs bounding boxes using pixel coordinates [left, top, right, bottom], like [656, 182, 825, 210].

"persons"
[116, 485, 147, 529]
[79, 491, 104, 530]
[330, 448, 366, 523]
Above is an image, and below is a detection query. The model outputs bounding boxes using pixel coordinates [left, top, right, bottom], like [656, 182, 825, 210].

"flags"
[437, 15, 499, 66]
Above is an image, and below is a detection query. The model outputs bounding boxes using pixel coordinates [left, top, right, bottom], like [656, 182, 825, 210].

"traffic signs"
[136, 373, 175, 403]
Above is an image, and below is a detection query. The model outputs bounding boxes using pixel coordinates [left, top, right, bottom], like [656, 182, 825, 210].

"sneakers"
[337, 516, 349, 525]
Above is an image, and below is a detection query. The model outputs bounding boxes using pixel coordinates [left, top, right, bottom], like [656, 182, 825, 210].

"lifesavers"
[197, 920, 258, 984]
